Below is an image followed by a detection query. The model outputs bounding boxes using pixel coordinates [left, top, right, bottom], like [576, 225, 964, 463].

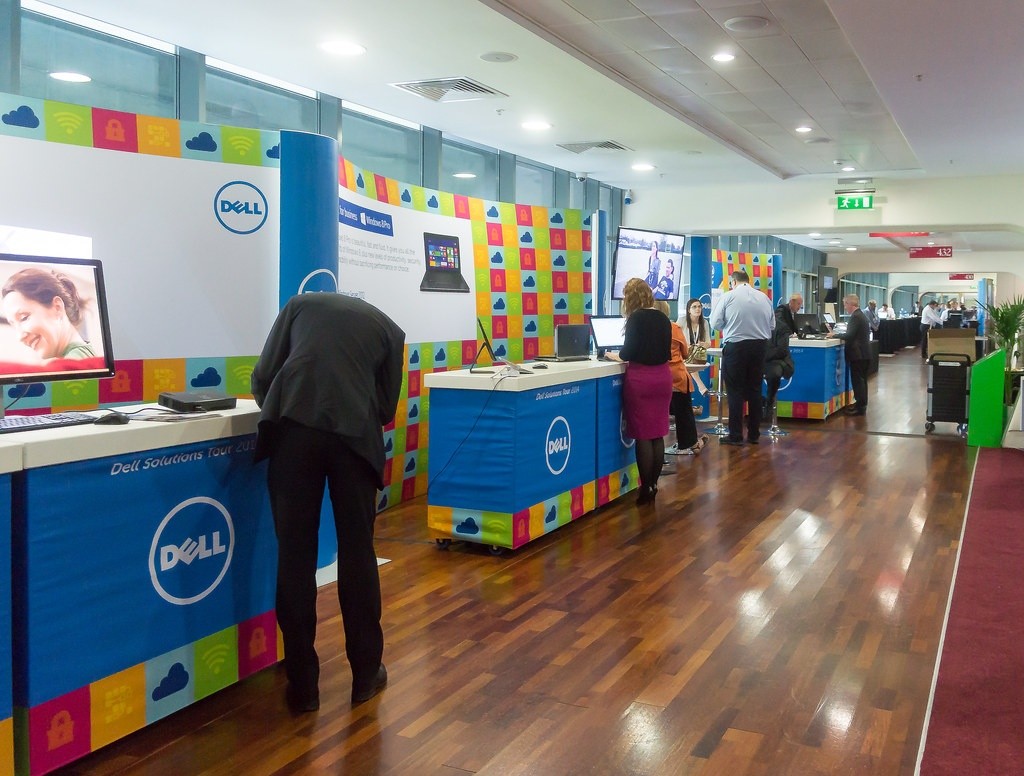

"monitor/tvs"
[610, 225, 686, 300]
[0, 252, 115, 387]
[469, 319, 497, 374]
[795, 313, 836, 339]
[590, 315, 627, 358]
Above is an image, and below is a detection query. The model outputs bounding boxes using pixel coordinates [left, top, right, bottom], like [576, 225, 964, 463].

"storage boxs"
[927, 328, 976, 363]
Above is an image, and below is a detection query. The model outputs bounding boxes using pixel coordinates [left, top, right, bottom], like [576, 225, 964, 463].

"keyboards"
[520, 372, 534, 374]
[0, 412, 98, 434]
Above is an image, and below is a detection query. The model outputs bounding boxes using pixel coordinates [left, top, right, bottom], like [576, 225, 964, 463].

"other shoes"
[762, 400, 774, 419]
[748, 436, 759, 444]
[843, 404, 865, 415]
[719, 435, 744, 445]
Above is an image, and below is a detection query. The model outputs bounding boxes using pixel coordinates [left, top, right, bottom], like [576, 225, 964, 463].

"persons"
[652, 300, 711, 455]
[919, 298, 967, 360]
[604, 276, 674, 504]
[650, 259, 674, 299]
[2, 267, 98, 360]
[760, 317, 794, 411]
[249, 291, 405, 718]
[708, 269, 776, 447]
[773, 293, 804, 338]
[642, 240, 660, 290]
[862, 298, 880, 340]
[676, 297, 711, 396]
[878, 304, 896, 320]
[829, 293, 874, 415]
[912, 301, 924, 316]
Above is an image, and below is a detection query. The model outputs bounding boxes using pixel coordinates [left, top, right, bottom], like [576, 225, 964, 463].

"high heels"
[636, 484, 658, 505]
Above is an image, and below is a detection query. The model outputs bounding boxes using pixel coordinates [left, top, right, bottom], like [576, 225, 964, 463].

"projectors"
[156, 388, 236, 413]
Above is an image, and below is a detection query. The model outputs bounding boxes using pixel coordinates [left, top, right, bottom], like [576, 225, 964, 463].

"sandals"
[692, 435, 709, 455]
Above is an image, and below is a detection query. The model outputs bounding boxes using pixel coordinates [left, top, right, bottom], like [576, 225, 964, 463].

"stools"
[760, 376, 794, 438]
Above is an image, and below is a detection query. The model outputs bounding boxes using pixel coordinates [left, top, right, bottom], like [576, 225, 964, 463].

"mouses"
[532, 363, 548, 369]
[94, 412, 130, 425]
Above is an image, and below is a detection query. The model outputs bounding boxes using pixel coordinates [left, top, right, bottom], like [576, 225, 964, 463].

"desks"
[874, 316, 922, 356]
[702, 347, 727, 434]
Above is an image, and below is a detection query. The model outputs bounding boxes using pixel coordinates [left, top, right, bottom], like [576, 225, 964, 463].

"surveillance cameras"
[576, 173, 588, 182]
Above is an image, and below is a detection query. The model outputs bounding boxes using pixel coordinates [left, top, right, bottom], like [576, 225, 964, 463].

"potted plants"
[969, 295, 1024, 421]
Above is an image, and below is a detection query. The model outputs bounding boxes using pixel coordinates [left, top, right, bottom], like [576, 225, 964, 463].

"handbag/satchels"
[684, 345, 707, 364]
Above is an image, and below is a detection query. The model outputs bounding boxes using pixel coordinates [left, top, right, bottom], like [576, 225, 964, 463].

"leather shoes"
[352, 663, 387, 701]
[286, 683, 320, 711]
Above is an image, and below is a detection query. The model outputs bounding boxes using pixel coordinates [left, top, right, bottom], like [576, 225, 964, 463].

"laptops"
[419, 232, 470, 292]
[533, 324, 591, 362]
